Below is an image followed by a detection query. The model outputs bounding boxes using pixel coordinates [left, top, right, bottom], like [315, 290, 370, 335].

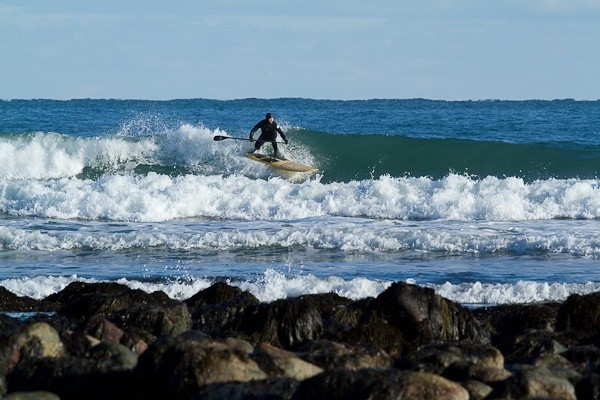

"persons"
[249, 112, 288, 159]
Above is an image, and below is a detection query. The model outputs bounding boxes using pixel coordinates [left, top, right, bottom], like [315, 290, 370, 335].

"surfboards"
[245, 150, 319, 172]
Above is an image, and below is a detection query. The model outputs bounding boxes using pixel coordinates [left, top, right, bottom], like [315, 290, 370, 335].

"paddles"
[214, 136, 290, 144]
